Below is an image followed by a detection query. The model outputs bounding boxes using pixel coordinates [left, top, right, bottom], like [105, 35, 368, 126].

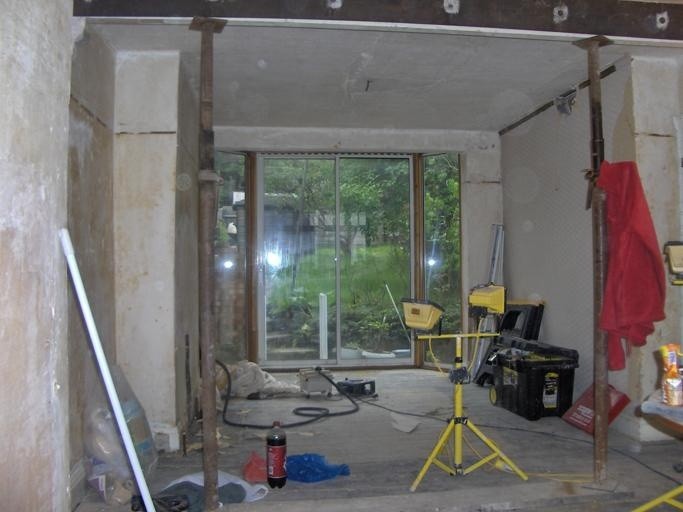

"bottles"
[265, 419, 287, 489]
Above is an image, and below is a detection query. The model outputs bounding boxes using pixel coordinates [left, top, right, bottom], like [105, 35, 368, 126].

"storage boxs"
[490, 348, 581, 421]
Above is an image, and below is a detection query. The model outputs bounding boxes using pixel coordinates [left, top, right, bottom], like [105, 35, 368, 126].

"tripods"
[409, 332, 528, 492]
